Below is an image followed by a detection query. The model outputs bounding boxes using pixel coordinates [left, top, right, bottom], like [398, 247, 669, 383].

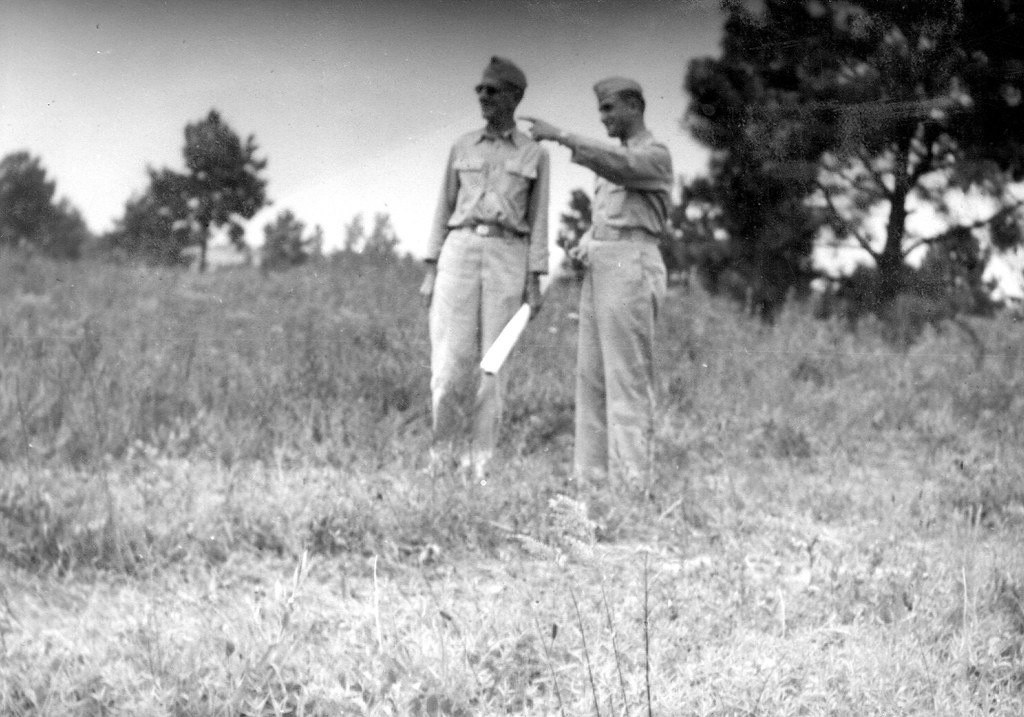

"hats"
[484, 56, 527, 90]
[592, 76, 642, 101]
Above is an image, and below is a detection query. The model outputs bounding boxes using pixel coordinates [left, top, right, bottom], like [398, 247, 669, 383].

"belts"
[472, 223, 504, 237]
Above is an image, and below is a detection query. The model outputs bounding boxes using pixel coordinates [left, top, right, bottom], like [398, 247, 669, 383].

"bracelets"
[560, 130, 568, 143]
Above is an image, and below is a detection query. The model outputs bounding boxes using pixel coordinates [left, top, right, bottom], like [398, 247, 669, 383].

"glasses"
[475, 84, 498, 95]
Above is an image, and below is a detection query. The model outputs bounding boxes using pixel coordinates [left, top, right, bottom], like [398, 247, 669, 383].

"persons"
[518, 76, 672, 491]
[421, 56, 549, 467]
